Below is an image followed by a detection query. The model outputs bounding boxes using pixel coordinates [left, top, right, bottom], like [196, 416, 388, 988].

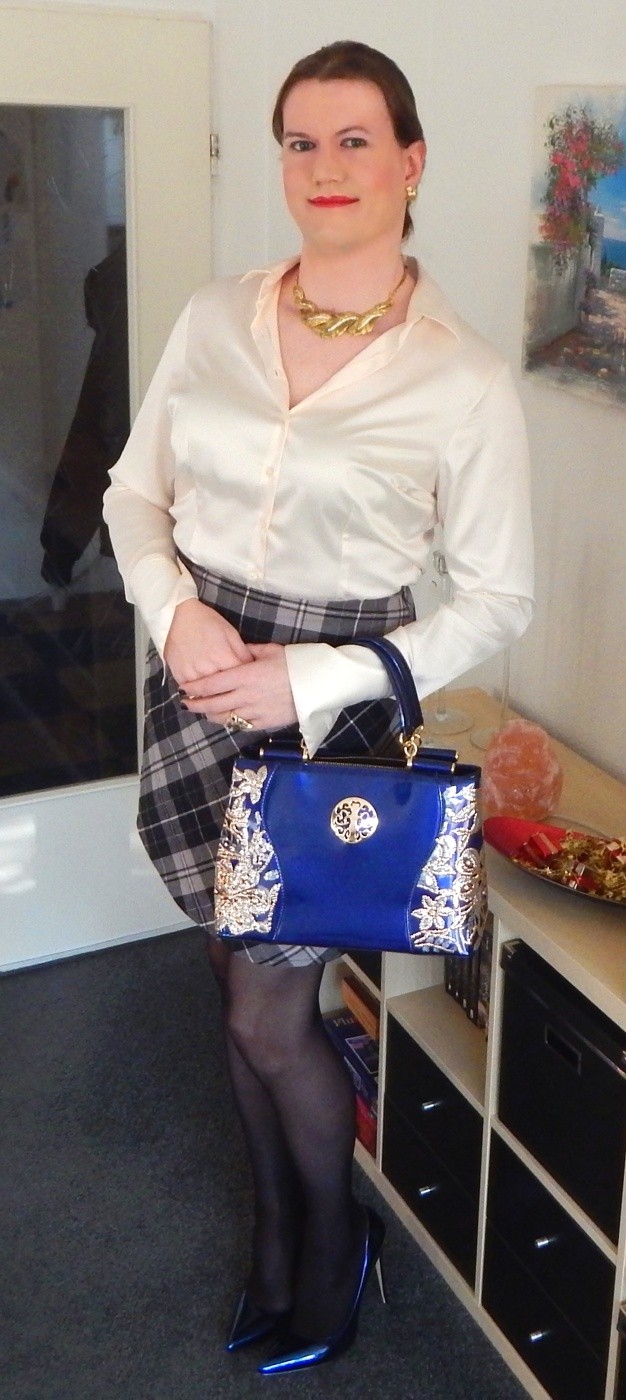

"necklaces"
[293, 264, 408, 338]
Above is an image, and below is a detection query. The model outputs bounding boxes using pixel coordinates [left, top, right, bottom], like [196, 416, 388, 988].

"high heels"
[257, 1203, 387, 1375]
[226, 1272, 294, 1351]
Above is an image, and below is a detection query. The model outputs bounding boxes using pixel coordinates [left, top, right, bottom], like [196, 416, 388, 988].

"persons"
[104, 40, 533, 1376]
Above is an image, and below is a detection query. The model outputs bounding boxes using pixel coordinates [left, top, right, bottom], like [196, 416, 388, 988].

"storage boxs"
[498, 939, 626, 1247]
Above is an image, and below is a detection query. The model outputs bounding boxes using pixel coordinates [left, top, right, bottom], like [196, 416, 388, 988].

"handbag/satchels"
[214, 635, 487, 956]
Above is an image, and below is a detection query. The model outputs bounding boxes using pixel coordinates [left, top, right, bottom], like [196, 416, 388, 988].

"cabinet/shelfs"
[316, 688, 626, 1399]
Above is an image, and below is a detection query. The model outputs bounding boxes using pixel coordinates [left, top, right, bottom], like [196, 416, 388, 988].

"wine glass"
[471, 643, 513, 750]
[421, 687, 479, 734]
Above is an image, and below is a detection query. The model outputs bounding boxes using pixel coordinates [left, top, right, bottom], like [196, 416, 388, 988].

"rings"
[189, 695, 196, 700]
[224, 709, 253, 730]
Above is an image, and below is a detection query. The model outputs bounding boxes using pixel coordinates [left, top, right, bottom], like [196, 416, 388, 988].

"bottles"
[479, 719, 562, 821]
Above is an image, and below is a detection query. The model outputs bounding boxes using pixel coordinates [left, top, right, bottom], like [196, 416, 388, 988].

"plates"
[482, 816, 626, 905]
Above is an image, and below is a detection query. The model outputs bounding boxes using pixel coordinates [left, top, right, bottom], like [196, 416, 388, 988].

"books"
[322, 976, 381, 1158]
[444, 907, 495, 1040]
